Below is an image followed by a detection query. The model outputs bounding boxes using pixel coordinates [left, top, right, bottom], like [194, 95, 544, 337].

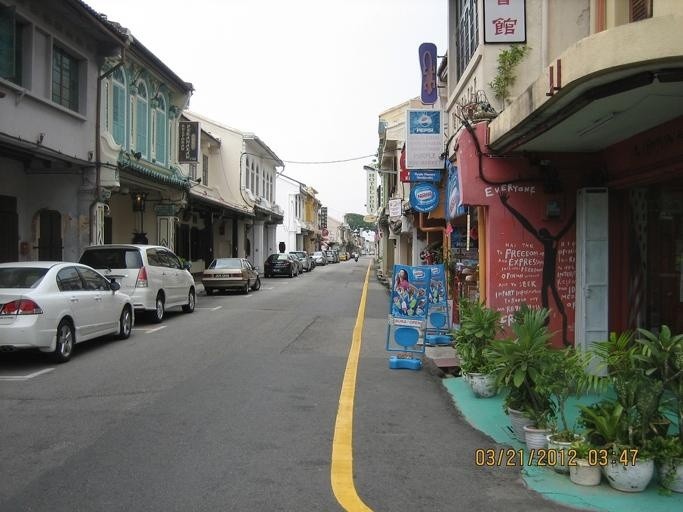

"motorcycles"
[354, 255, 359, 262]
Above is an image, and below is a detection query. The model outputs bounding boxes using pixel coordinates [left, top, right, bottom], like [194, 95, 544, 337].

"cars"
[0, 243, 261, 364]
[264, 250, 356, 278]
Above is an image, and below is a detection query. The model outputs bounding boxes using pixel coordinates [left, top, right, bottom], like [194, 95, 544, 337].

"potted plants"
[455, 296, 683, 493]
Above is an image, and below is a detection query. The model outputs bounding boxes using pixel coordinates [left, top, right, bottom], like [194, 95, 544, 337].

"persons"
[392, 268, 419, 295]
[354, 252, 359, 260]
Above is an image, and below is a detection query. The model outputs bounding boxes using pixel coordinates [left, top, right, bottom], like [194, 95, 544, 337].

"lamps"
[88, 150, 93, 159]
[37, 133, 45, 143]
[188, 177, 201, 188]
[136, 152, 141, 158]
